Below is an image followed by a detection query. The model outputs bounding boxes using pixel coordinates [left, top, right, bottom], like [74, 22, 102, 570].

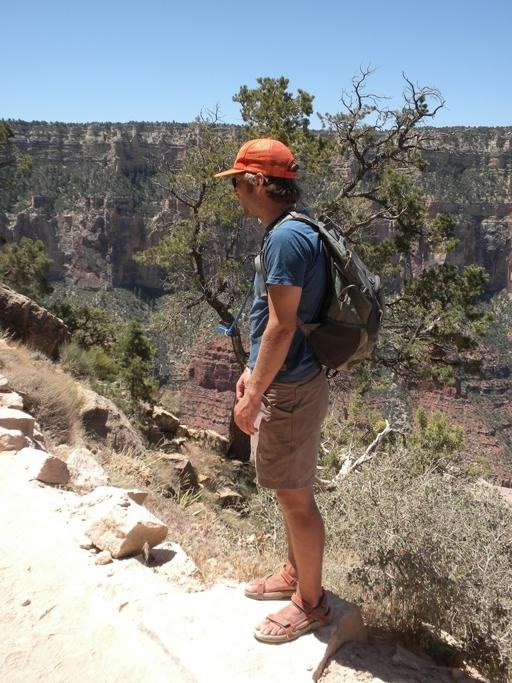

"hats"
[214, 139, 298, 178]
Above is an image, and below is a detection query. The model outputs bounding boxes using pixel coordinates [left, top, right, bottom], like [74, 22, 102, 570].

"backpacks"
[262, 211, 386, 374]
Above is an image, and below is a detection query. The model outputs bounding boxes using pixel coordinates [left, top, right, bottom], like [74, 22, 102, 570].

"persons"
[214, 138, 334, 643]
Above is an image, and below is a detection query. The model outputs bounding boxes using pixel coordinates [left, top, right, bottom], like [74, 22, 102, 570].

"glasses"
[232, 178, 253, 187]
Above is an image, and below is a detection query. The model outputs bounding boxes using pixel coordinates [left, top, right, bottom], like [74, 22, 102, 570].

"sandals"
[244, 565, 297, 600]
[254, 587, 334, 643]
